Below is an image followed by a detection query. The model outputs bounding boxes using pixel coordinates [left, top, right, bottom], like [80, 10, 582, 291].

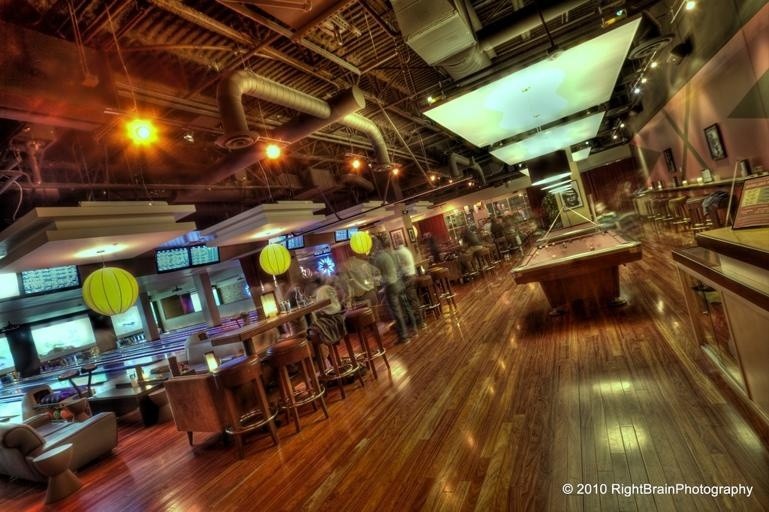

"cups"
[282, 300, 292, 315]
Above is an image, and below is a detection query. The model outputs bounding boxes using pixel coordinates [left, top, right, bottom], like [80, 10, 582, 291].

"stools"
[31, 442, 84, 504]
[637, 197, 713, 240]
[213, 355, 279, 460]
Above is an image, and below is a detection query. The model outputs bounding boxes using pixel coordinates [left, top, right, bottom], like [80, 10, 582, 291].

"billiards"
[545, 245, 548, 248]
[590, 247, 594, 251]
[539, 246, 542, 249]
[563, 244, 567, 248]
[552, 255, 556, 258]
[552, 242, 555, 245]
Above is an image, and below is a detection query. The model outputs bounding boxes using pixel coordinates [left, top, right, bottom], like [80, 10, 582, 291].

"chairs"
[163, 373, 233, 451]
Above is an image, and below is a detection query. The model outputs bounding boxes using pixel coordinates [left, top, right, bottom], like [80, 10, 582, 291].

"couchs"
[0, 411, 117, 490]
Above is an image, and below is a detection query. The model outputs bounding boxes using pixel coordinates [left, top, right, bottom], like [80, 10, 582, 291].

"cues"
[568, 208, 597, 225]
[543, 208, 564, 238]
[725, 160, 737, 227]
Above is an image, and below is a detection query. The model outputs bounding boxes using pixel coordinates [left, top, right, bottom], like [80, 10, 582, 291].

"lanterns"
[259, 244, 291, 277]
[82, 266, 139, 318]
[349, 232, 373, 255]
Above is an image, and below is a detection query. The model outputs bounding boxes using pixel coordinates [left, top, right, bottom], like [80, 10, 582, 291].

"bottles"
[294, 288, 305, 309]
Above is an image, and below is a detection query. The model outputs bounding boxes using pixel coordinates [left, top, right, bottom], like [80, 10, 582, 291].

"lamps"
[414, 0, 657, 195]
[345, 220, 372, 253]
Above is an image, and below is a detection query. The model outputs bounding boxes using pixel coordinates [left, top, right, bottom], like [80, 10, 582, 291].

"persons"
[369, 240, 419, 345]
[326, 246, 383, 325]
[308, 275, 342, 373]
[423, 231, 440, 261]
[457, 210, 524, 276]
[282, 278, 311, 333]
[393, 237, 428, 328]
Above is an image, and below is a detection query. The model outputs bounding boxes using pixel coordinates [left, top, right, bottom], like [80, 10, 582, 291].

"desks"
[648, 172, 768, 225]
[510, 227, 642, 316]
[536, 220, 605, 246]
[1, 236, 496, 429]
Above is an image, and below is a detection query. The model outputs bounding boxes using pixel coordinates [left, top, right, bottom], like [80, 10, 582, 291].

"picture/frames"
[704, 123, 728, 161]
[559, 179, 584, 211]
[663, 148, 676, 173]
